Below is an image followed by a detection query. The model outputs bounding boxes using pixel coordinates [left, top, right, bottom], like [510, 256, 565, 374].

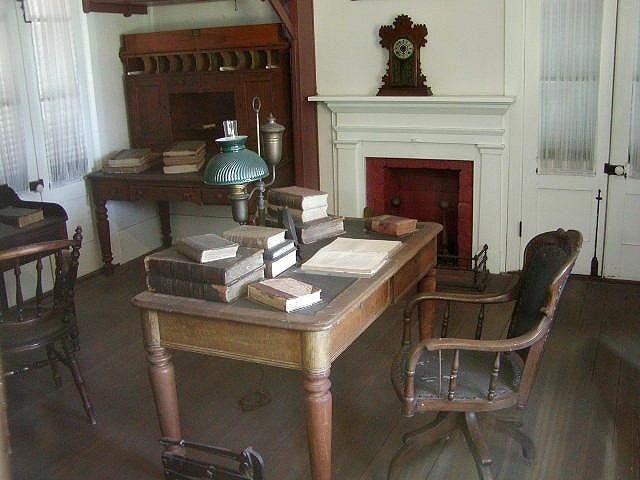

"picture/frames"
[1, 184, 69, 312]
[85, 149, 291, 275]
[133, 217, 445, 480]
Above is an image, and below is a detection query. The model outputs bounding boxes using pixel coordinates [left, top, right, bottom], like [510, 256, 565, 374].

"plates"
[118, 23, 290, 149]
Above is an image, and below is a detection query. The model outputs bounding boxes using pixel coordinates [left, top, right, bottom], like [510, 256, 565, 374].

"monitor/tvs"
[145, 185, 420, 313]
[102, 139, 206, 174]
[0, 206, 44, 228]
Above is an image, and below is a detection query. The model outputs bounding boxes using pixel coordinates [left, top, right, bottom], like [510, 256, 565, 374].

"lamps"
[387, 227, 582, 479]
[0, 226, 97, 456]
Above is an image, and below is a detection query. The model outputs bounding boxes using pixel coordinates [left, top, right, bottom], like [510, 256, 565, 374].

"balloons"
[377, 13, 433, 96]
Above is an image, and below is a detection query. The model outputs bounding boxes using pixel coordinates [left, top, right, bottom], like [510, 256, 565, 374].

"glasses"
[200, 95, 283, 223]
